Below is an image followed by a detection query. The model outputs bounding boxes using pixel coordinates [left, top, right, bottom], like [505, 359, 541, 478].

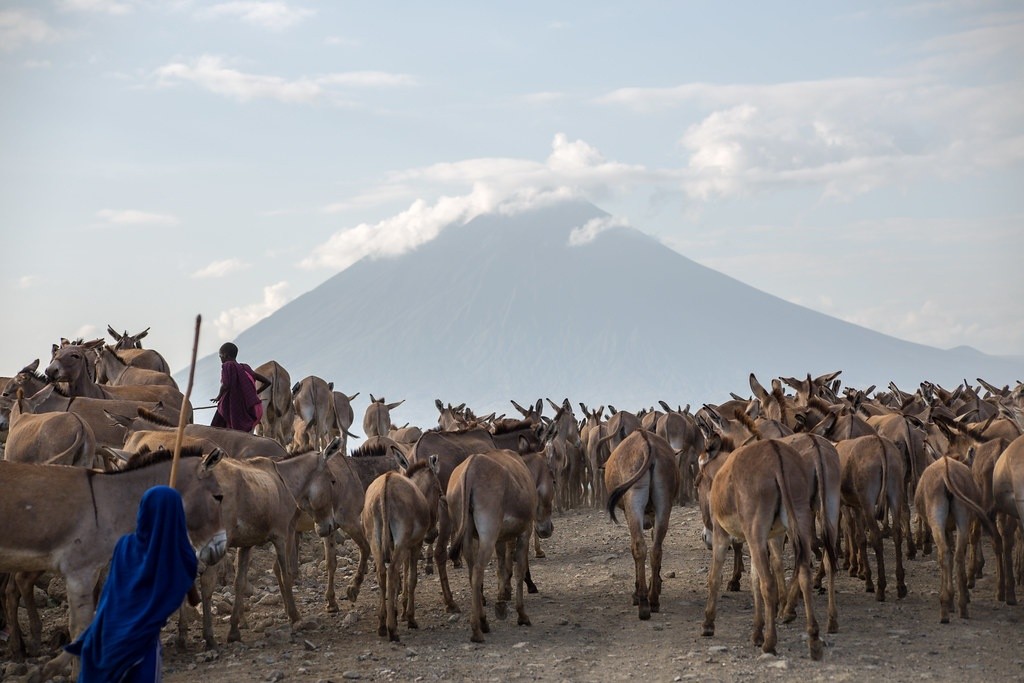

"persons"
[208, 342, 272, 431]
[65, 484, 198, 683]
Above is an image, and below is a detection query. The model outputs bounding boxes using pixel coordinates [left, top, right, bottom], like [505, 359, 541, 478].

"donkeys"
[1, 326, 1024, 659]
